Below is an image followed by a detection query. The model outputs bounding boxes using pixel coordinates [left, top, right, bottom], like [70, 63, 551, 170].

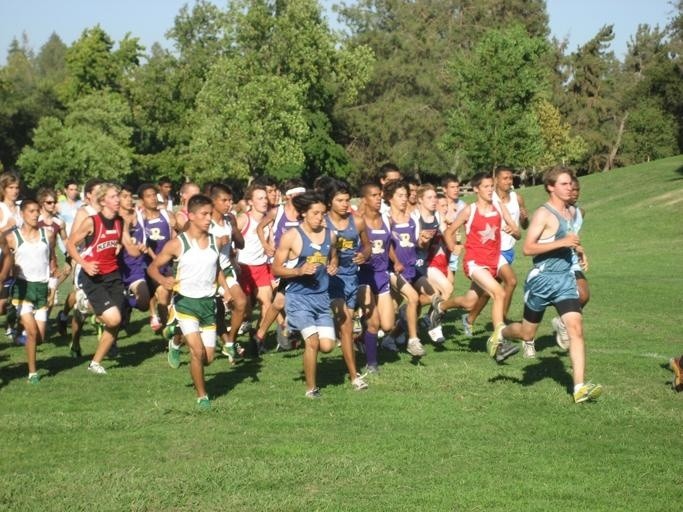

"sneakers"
[198, 394, 211, 409]
[360, 361, 377, 380]
[351, 306, 364, 337]
[305, 387, 323, 399]
[167, 340, 181, 369]
[553, 317, 571, 350]
[486, 322, 504, 357]
[431, 298, 445, 328]
[88, 361, 105, 375]
[398, 331, 407, 345]
[56, 314, 68, 338]
[463, 314, 476, 339]
[71, 346, 82, 362]
[236, 341, 248, 357]
[283, 338, 302, 349]
[521, 340, 536, 361]
[163, 322, 175, 340]
[237, 318, 254, 337]
[384, 334, 399, 353]
[223, 345, 238, 367]
[351, 379, 371, 391]
[354, 339, 365, 355]
[428, 326, 448, 344]
[406, 337, 427, 358]
[149, 314, 162, 331]
[574, 384, 604, 406]
[109, 341, 120, 359]
[91, 315, 100, 327]
[494, 338, 520, 363]
[26, 374, 40, 385]
[251, 332, 263, 349]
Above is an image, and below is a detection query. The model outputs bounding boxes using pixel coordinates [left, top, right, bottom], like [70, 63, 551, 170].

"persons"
[521, 175, 591, 360]
[486, 166, 603, 404]
[431, 166, 530, 362]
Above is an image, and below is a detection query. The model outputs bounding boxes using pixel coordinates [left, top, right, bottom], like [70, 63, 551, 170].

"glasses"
[45, 200, 59, 206]
